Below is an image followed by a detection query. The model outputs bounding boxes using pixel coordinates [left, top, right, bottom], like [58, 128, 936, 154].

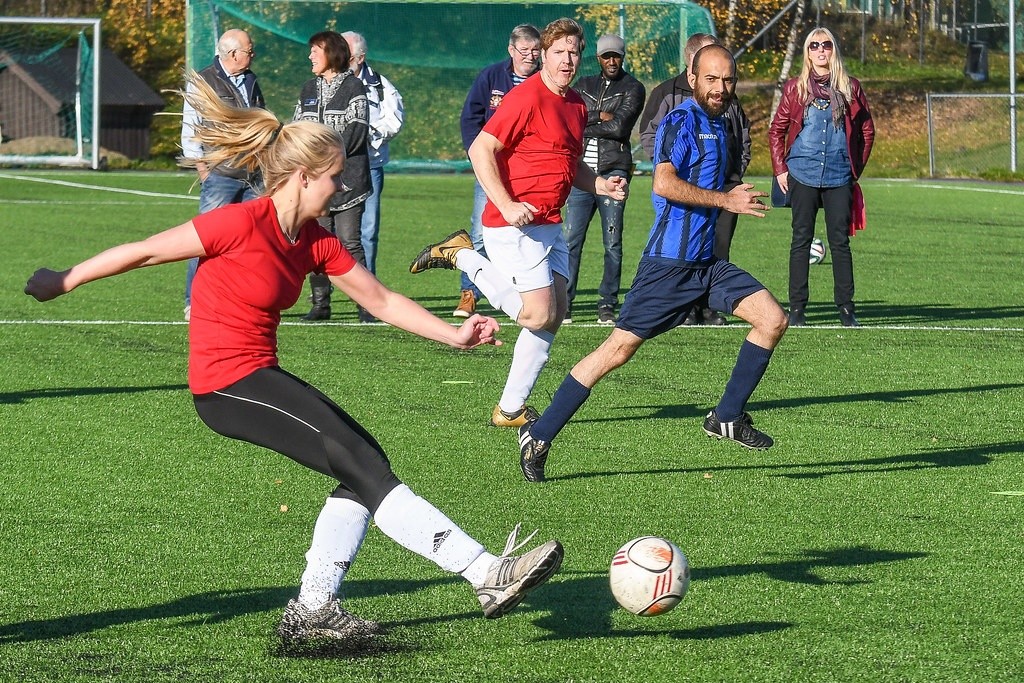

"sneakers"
[598, 298, 616, 325]
[491, 404, 541, 427]
[474, 521, 564, 618]
[454, 288, 476, 318]
[517, 420, 552, 482]
[562, 312, 572, 324]
[409, 230, 474, 274]
[278, 600, 389, 644]
[703, 407, 774, 450]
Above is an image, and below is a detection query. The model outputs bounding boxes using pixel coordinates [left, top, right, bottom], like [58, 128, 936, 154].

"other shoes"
[789, 310, 806, 327]
[683, 308, 696, 325]
[839, 301, 859, 326]
[700, 309, 727, 325]
[301, 308, 331, 320]
[184, 308, 191, 321]
[358, 307, 380, 322]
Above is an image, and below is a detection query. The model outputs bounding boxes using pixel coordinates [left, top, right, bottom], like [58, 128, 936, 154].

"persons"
[632, 32, 752, 325]
[410, 16, 631, 427]
[24, 67, 564, 640]
[518, 44, 792, 482]
[451, 25, 540, 319]
[181, 31, 403, 323]
[561, 32, 647, 324]
[769, 28, 876, 326]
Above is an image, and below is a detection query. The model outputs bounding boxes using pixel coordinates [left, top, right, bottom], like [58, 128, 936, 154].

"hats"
[597, 34, 625, 56]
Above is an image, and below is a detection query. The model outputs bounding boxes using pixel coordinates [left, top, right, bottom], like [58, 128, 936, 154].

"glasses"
[514, 48, 541, 58]
[810, 41, 833, 50]
[226, 49, 255, 59]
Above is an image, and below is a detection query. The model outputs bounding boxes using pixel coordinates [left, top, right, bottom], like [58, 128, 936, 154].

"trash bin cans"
[965, 40, 988, 82]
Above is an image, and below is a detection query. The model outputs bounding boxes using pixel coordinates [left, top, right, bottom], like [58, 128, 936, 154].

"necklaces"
[811, 98, 831, 110]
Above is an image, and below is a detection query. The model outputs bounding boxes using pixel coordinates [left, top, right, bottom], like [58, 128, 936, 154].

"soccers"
[608, 535, 691, 617]
